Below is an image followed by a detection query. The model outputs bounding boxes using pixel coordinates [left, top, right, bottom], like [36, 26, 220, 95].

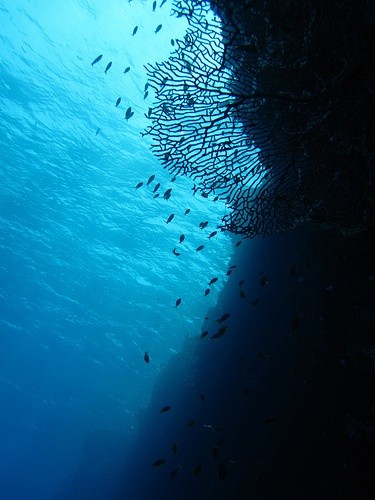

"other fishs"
[88, 0, 375, 500]
